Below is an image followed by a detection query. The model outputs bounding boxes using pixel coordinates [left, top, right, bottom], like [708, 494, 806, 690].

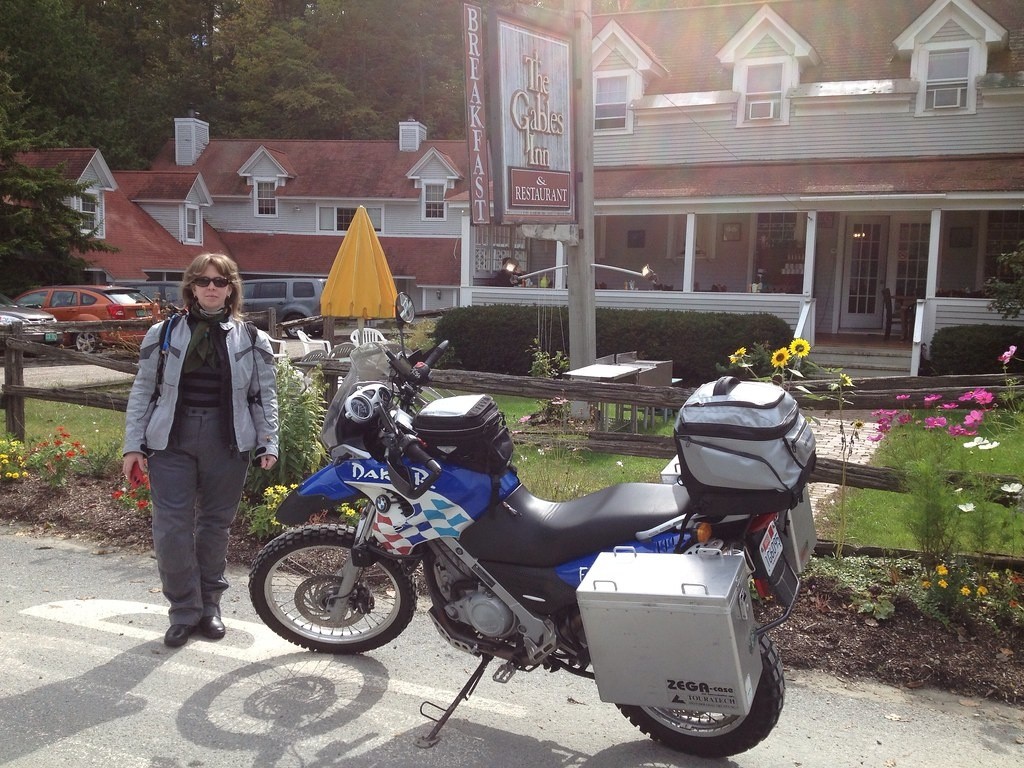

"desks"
[889, 295, 924, 340]
[294, 356, 351, 421]
[561, 364, 640, 434]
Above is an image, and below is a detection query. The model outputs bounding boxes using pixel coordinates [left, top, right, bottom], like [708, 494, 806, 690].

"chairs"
[55, 294, 69, 306]
[882, 288, 916, 341]
[595, 351, 673, 431]
[262, 327, 456, 408]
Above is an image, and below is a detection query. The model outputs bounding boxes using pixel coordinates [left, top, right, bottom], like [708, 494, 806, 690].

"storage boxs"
[660, 453, 817, 574]
[575, 546, 763, 716]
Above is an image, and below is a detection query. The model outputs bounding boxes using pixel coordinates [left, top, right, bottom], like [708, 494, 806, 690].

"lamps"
[295, 205, 301, 211]
[436, 290, 441, 300]
[591, 264, 657, 283]
[502, 258, 568, 285]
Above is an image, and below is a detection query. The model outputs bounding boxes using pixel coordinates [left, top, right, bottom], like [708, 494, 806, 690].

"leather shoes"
[199, 616, 226, 639]
[164, 624, 199, 646]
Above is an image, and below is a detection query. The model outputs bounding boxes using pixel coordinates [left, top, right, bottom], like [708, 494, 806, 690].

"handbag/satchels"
[674, 374, 818, 520]
[414, 392, 516, 478]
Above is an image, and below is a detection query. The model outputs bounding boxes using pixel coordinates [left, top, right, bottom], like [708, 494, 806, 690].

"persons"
[122, 253, 279, 647]
[492, 257, 527, 287]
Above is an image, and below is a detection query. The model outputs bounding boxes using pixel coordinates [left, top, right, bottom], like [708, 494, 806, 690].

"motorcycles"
[245, 291, 818, 760]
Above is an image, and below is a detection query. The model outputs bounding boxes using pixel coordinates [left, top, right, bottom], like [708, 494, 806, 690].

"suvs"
[238, 278, 335, 338]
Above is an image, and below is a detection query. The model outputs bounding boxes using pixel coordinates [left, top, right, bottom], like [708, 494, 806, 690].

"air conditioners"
[934, 89, 960, 108]
[749, 102, 776, 119]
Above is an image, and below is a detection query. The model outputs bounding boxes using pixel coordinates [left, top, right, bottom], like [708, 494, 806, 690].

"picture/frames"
[949, 227, 973, 247]
[722, 223, 741, 241]
[628, 230, 645, 249]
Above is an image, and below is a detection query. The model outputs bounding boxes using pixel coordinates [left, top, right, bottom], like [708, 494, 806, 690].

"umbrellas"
[320, 206, 399, 346]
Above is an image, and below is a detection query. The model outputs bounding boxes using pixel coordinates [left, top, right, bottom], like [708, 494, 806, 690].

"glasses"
[193, 276, 233, 287]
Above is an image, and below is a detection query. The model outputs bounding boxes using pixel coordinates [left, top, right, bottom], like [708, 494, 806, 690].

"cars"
[106, 280, 188, 309]
[0, 293, 59, 359]
[7, 284, 162, 355]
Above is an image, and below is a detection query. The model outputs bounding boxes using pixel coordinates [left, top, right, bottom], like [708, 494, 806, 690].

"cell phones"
[130, 461, 146, 489]
